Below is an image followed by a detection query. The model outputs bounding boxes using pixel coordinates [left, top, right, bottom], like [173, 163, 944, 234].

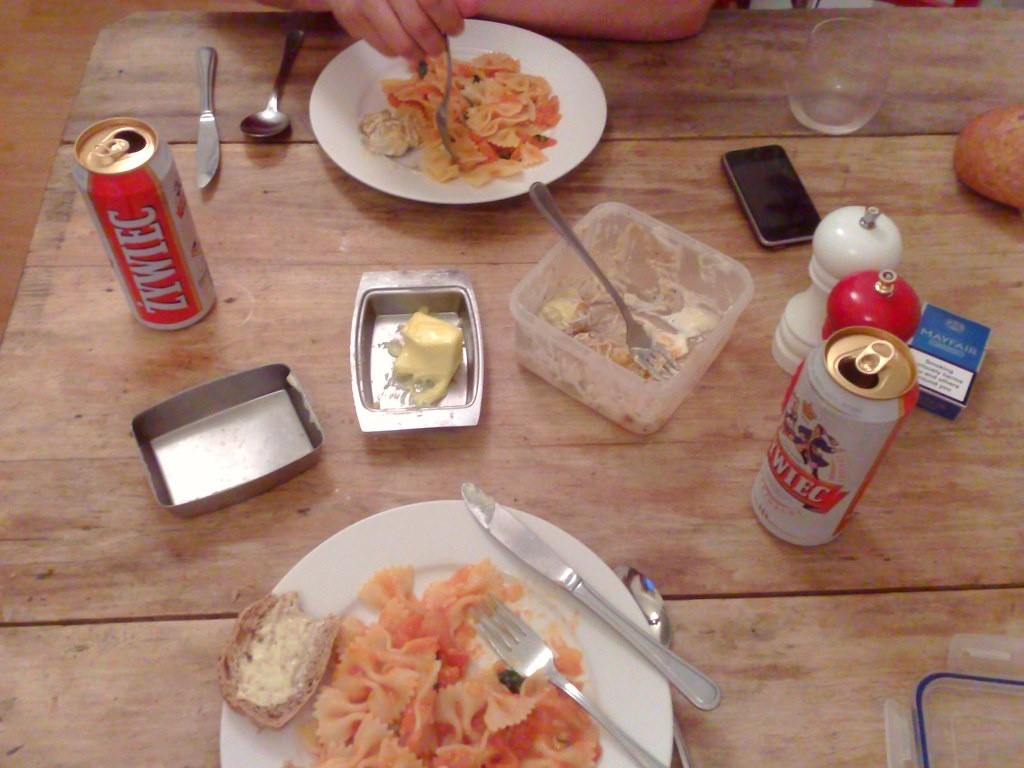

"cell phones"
[722, 144, 824, 248]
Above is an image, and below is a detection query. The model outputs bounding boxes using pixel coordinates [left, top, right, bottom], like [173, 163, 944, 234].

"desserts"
[393, 312, 462, 404]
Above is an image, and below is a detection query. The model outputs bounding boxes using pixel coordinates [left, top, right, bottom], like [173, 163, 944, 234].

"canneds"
[751, 325, 920, 547]
[69, 116, 216, 331]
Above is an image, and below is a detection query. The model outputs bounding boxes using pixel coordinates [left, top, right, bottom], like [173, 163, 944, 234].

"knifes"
[460, 481, 723, 714]
[193, 46, 222, 190]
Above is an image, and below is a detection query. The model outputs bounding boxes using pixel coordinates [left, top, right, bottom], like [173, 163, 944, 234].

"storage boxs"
[130, 363, 325, 519]
[347, 266, 487, 435]
[507, 200, 755, 434]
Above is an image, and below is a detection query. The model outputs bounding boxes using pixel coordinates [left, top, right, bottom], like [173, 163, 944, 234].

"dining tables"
[0, 6, 1023, 768]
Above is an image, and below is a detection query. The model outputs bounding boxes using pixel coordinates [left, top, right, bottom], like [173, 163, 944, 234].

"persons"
[226, 0, 713, 58]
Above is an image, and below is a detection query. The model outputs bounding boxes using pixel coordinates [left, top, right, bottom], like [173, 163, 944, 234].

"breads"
[218, 591, 340, 729]
[952, 99, 1024, 214]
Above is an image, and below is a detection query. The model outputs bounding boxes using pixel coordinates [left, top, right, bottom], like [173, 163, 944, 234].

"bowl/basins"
[507, 199, 757, 434]
[349, 263, 486, 433]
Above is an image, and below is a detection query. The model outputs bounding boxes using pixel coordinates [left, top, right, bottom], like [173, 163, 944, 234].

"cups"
[789, 16, 896, 138]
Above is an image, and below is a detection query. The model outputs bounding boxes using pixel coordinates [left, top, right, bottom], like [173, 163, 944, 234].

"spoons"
[611, 565, 694, 768]
[239, 26, 304, 138]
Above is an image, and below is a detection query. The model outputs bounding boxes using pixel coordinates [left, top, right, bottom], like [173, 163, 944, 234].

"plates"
[219, 500, 674, 768]
[310, 19, 609, 206]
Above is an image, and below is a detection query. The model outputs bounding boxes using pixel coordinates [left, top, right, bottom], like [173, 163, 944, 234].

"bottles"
[771, 204, 904, 375]
[782, 270, 920, 415]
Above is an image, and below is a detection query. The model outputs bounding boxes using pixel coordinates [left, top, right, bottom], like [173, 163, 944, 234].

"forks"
[528, 179, 682, 384]
[435, 30, 460, 163]
[468, 593, 665, 768]
